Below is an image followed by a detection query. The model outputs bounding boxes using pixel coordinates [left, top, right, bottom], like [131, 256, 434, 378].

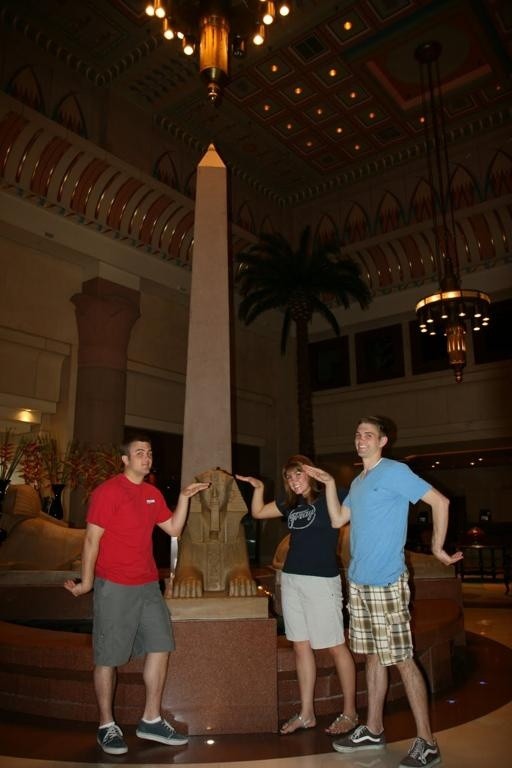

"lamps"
[143, 0, 290, 104]
[413, 39, 492, 384]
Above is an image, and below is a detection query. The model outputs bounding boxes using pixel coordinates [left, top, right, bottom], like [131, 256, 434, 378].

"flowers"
[0, 424, 30, 480]
[20, 432, 123, 506]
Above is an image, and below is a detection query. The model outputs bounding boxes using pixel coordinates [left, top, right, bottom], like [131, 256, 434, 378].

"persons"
[172, 467, 258, 597]
[300, 416, 464, 768]
[63, 435, 210, 756]
[235, 455, 359, 737]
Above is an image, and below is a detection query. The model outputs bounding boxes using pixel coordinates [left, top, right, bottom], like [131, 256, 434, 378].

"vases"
[47, 482, 66, 520]
[0, 478, 11, 505]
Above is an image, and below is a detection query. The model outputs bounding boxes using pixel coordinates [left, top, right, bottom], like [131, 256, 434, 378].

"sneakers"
[135, 716, 189, 746]
[398, 735, 442, 768]
[97, 724, 130, 755]
[333, 725, 386, 753]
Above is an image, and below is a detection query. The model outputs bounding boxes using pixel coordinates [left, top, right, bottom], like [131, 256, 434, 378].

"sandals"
[279, 714, 317, 734]
[325, 712, 359, 737]
